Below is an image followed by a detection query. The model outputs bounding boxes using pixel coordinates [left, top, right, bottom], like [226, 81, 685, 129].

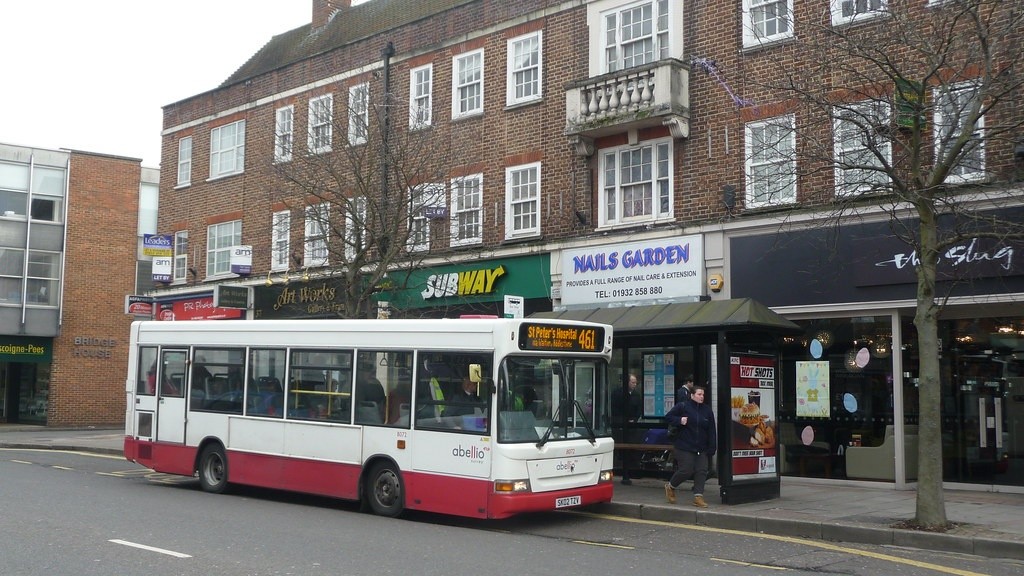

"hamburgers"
[738, 404, 761, 425]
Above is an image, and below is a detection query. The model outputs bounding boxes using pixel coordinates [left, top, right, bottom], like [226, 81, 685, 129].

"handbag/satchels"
[665, 402, 685, 443]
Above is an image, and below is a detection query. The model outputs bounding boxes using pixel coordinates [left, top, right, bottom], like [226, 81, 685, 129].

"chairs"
[358, 401, 381, 425]
[171, 373, 295, 414]
[430, 377, 446, 416]
[400, 404, 410, 418]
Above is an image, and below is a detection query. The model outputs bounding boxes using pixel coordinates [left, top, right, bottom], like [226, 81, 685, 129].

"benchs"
[615, 443, 674, 451]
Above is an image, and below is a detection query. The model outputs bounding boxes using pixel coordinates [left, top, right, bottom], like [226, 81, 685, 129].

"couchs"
[779, 423, 831, 473]
[846, 435, 918, 480]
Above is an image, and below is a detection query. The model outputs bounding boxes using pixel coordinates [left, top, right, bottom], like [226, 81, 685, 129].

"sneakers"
[664, 482, 678, 504]
[692, 496, 708, 508]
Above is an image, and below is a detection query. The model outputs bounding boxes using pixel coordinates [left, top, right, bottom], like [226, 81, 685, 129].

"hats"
[684, 374, 693, 382]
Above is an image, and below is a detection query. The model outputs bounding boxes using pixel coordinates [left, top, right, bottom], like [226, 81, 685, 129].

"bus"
[124, 313, 614, 521]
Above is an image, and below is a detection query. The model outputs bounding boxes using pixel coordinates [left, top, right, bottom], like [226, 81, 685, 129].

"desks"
[790, 452, 831, 475]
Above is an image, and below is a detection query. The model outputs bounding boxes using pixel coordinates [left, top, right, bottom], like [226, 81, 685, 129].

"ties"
[469, 394, 474, 398]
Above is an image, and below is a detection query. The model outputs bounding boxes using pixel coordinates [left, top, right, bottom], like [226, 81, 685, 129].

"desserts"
[751, 422, 773, 446]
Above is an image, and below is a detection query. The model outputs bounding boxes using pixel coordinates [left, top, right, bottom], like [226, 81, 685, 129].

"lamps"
[284, 268, 299, 286]
[815, 328, 835, 349]
[871, 323, 891, 358]
[844, 320, 862, 373]
[266, 270, 284, 287]
[301, 265, 323, 283]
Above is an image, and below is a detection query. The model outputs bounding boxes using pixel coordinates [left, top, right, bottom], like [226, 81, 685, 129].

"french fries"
[732, 395, 745, 409]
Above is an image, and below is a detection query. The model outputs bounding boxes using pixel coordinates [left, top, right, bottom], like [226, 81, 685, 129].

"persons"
[146, 357, 178, 395]
[228, 360, 256, 403]
[192, 355, 221, 392]
[299, 358, 325, 418]
[664, 386, 716, 508]
[440, 372, 483, 430]
[335, 361, 384, 424]
[611, 373, 642, 485]
[331, 379, 338, 392]
[676, 375, 695, 401]
